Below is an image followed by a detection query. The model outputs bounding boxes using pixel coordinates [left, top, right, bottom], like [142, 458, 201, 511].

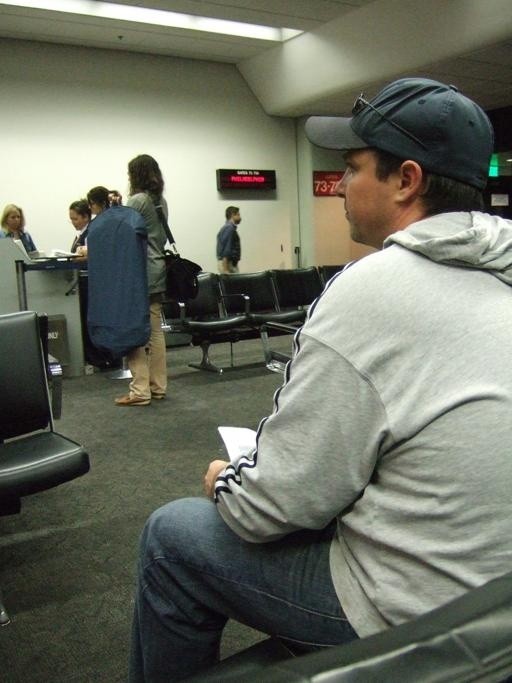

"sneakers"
[115, 392, 165, 405]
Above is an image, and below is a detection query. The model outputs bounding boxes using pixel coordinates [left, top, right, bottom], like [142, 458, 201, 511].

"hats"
[304, 76, 495, 190]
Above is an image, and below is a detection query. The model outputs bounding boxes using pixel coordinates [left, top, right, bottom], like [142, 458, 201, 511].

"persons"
[125, 75, 512, 682]
[69, 198, 106, 374]
[75, 184, 125, 374]
[1, 203, 42, 259]
[107, 153, 171, 407]
[215, 204, 243, 275]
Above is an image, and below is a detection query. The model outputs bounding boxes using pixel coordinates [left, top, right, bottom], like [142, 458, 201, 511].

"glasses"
[351, 93, 427, 149]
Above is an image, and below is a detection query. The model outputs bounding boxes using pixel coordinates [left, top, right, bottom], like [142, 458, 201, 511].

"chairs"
[0, 309, 90, 522]
[177, 566, 511, 683]
[160, 264, 344, 375]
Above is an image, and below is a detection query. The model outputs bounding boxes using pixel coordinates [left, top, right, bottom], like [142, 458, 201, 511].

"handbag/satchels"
[163, 249, 202, 302]
